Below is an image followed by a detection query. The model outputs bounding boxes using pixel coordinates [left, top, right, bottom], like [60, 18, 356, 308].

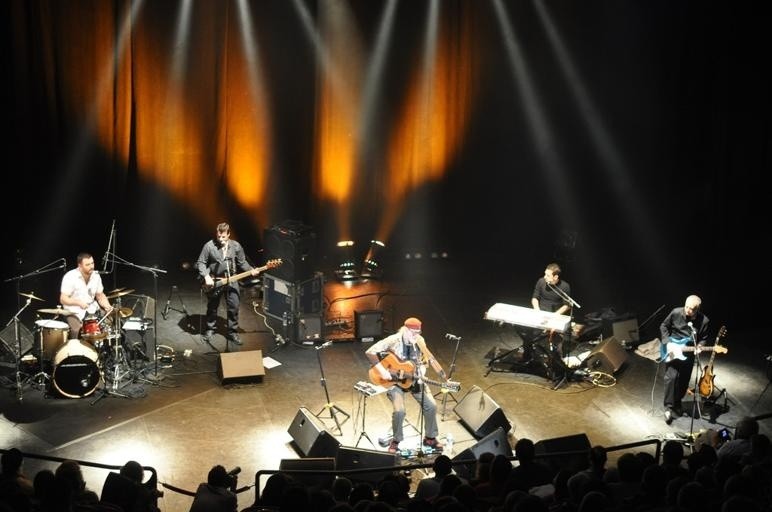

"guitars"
[368, 348, 461, 395]
[202, 256, 282, 295]
[701, 326, 727, 401]
[663, 339, 726, 362]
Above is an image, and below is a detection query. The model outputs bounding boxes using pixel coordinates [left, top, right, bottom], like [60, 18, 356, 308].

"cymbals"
[103, 287, 132, 301]
[20, 291, 45, 305]
[106, 307, 132, 318]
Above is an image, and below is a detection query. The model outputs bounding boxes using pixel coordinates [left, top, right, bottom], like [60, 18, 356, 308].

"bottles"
[446, 431, 454, 454]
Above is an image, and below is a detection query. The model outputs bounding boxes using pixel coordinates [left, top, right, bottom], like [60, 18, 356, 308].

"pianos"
[485, 302, 574, 335]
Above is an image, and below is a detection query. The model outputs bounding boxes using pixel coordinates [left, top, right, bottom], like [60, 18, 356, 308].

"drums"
[81, 317, 105, 336]
[52, 338, 101, 399]
[33, 319, 68, 360]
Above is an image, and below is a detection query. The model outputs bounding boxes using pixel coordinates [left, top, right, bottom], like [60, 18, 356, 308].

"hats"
[404, 317, 422, 330]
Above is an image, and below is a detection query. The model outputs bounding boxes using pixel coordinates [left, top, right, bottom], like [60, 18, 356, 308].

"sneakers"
[423, 437, 443, 451]
[225, 333, 243, 345]
[389, 442, 398, 454]
[517, 354, 533, 361]
[664, 410, 672, 423]
[203, 329, 215, 341]
[676, 407, 683, 416]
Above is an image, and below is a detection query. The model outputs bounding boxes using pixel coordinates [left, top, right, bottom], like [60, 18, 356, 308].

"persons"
[659, 295, 711, 424]
[513, 263, 572, 358]
[189, 465, 237, 511]
[59, 253, 114, 340]
[258, 416, 772, 512]
[0, 448, 158, 512]
[364, 318, 452, 455]
[196, 223, 259, 345]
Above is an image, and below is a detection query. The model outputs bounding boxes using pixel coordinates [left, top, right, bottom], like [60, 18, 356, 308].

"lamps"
[334, 240, 385, 281]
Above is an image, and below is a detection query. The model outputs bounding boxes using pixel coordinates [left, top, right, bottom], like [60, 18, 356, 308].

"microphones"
[547, 281, 554, 286]
[316, 341, 332, 350]
[221, 240, 227, 251]
[63, 259, 66, 272]
[687, 321, 697, 335]
[101, 253, 106, 265]
[445, 334, 459, 341]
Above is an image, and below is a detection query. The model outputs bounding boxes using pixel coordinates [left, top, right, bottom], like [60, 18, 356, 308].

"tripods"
[161, 281, 189, 320]
[552, 283, 581, 391]
[315, 346, 350, 436]
[119, 344, 154, 390]
[25, 327, 50, 392]
[664, 331, 703, 454]
[90, 351, 129, 406]
[400, 382, 442, 466]
[355, 392, 375, 448]
[432, 337, 461, 421]
[0, 317, 36, 401]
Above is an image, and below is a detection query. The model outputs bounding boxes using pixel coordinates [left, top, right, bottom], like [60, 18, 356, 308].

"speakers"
[121, 294, 158, 320]
[216, 349, 266, 386]
[287, 407, 342, 457]
[121, 328, 154, 362]
[534, 433, 593, 468]
[0, 322, 35, 363]
[581, 336, 629, 376]
[336, 447, 401, 490]
[279, 460, 336, 490]
[451, 426, 514, 481]
[453, 384, 512, 438]
[602, 315, 643, 352]
[354, 309, 384, 341]
[263, 222, 310, 272]
[294, 313, 325, 345]
